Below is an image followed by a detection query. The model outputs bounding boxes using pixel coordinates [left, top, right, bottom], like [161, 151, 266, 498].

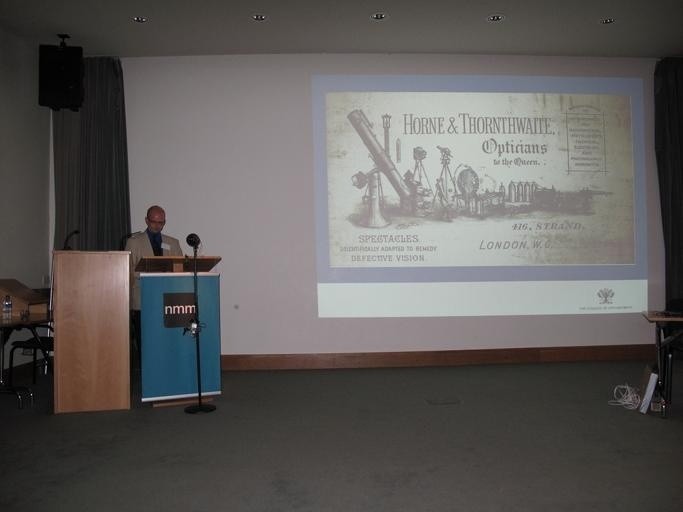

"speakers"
[36, 43, 85, 113]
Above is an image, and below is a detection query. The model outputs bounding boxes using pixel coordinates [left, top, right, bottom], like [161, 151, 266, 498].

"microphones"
[62, 229, 79, 251]
[185, 233, 200, 247]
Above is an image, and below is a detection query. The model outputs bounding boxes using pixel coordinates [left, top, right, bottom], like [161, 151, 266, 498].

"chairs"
[8, 325, 54, 388]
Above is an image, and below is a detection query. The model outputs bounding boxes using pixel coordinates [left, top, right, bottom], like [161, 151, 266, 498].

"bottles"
[3, 295, 12, 320]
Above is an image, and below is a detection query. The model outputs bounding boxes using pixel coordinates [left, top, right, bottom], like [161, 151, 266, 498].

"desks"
[0, 314, 54, 386]
[642, 310, 683, 418]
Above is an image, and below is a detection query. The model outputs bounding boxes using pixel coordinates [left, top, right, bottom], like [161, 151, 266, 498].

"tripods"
[432, 164, 459, 206]
[412, 159, 434, 197]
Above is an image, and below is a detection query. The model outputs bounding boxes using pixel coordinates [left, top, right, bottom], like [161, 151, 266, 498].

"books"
[637, 364, 658, 415]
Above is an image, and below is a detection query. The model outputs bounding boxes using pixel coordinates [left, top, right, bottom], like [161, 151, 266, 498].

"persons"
[123, 205, 183, 377]
